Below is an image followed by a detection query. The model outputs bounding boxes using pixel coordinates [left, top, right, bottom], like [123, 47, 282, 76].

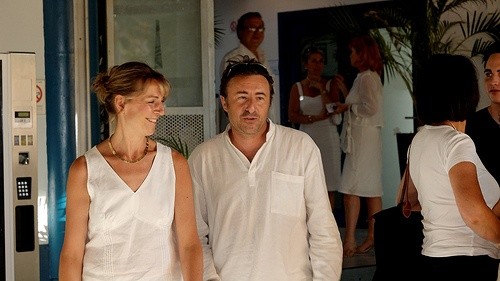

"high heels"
[343, 244, 358, 258]
[358, 244, 373, 254]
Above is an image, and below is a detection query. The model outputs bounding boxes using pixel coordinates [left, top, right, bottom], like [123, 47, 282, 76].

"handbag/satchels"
[372, 142, 425, 281]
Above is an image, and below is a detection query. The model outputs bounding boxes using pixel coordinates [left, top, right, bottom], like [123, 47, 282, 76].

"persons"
[396, 53, 499, 281]
[58, 62, 206, 281]
[221, 11, 276, 74]
[288, 34, 385, 258]
[185, 53, 344, 281]
[464, 42, 500, 185]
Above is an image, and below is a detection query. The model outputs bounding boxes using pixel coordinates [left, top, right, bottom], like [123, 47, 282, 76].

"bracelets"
[307, 113, 313, 123]
[322, 89, 328, 96]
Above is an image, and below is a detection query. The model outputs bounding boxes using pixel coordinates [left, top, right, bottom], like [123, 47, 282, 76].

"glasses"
[243, 27, 265, 32]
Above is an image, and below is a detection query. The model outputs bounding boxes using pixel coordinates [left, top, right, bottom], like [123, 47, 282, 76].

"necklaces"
[448, 120, 458, 131]
[109, 133, 149, 164]
